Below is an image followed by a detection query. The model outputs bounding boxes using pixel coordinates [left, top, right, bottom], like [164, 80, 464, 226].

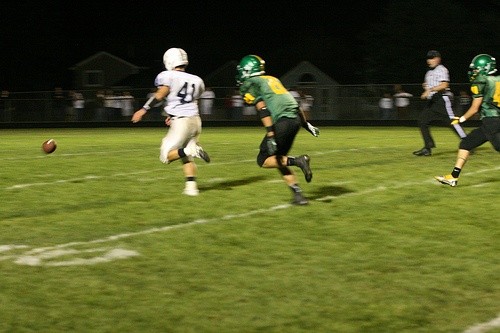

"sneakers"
[413, 148, 431, 156]
[182, 189, 199, 196]
[434, 174, 459, 187]
[188, 142, 211, 164]
[295, 154, 313, 183]
[291, 199, 308, 206]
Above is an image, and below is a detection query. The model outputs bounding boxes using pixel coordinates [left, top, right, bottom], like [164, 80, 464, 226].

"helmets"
[467, 54, 498, 83]
[163, 48, 188, 71]
[237, 55, 265, 81]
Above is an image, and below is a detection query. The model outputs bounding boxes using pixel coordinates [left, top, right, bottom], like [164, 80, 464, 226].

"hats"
[426, 50, 440, 60]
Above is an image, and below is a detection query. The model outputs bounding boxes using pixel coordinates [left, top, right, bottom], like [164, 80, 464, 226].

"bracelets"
[430, 88, 434, 92]
[460, 116, 465, 122]
[266, 124, 274, 132]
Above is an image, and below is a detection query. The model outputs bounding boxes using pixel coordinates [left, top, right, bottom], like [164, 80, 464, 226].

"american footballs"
[43, 139, 56, 154]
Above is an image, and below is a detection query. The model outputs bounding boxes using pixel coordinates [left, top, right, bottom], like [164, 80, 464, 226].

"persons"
[147, 88, 163, 119]
[199, 86, 215, 118]
[235, 55, 320, 205]
[53, 87, 85, 121]
[435, 54, 500, 187]
[0, 90, 14, 122]
[379, 92, 393, 118]
[459, 89, 470, 116]
[393, 85, 413, 119]
[291, 90, 313, 121]
[131, 48, 210, 196]
[225, 93, 257, 119]
[97, 87, 134, 119]
[412, 50, 468, 156]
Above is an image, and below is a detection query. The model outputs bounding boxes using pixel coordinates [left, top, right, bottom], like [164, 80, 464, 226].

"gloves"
[266, 136, 277, 154]
[304, 121, 320, 137]
